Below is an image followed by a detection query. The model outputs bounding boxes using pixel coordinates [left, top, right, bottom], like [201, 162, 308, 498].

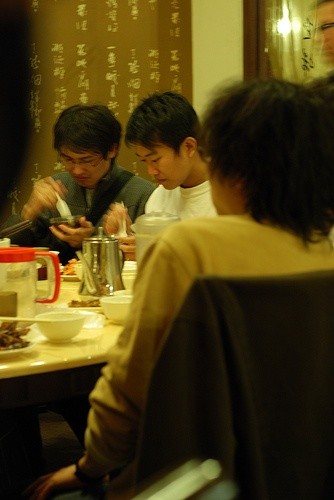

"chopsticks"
[0, 317, 50, 322]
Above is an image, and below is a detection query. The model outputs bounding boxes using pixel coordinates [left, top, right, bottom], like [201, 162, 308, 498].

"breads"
[63, 258, 78, 277]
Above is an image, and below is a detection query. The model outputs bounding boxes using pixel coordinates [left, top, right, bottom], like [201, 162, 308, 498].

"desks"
[0, 257, 137, 408]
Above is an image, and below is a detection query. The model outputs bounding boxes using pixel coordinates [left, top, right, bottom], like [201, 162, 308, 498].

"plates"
[0, 339, 39, 355]
[60, 275, 80, 282]
[54, 304, 104, 314]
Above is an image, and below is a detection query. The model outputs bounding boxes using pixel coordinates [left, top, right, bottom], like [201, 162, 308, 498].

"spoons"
[114, 201, 126, 233]
[54, 191, 71, 217]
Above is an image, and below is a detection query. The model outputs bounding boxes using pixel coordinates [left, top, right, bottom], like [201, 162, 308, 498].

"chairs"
[123, 274, 334, 500]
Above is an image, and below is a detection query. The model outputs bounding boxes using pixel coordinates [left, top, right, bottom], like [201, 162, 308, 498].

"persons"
[21, 103, 155, 266]
[101, 91, 218, 261]
[29, 78, 334, 500]
[307, 0, 334, 102]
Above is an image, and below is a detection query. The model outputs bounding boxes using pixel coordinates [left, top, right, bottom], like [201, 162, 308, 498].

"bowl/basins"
[49, 215, 84, 231]
[35, 312, 86, 343]
[99, 290, 134, 325]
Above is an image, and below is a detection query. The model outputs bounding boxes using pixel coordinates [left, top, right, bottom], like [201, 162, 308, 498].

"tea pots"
[75, 226, 125, 297]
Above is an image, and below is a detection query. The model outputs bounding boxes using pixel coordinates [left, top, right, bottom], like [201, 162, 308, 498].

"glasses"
[57, 152, 106, 172]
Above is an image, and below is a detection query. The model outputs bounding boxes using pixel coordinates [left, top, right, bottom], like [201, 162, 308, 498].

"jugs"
[0, 246, 60, 328]
[130, 213, 181, 267]
